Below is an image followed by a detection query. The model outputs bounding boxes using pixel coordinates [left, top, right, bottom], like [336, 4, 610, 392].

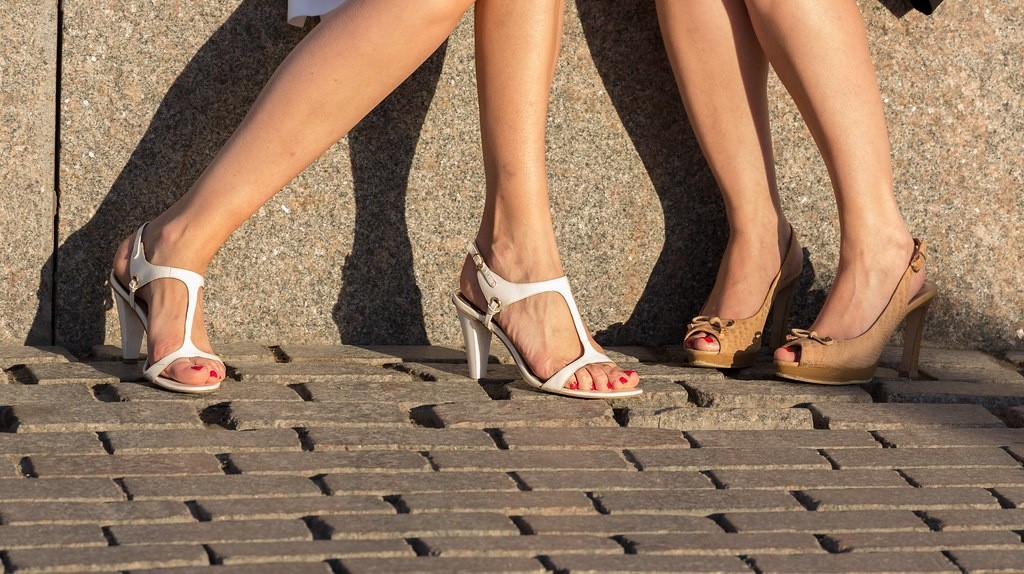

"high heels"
[110, 222, 227, 394]
[682, 224, 803, 369]
[452, 240, 644, 398]
[774, 236, 939, 385]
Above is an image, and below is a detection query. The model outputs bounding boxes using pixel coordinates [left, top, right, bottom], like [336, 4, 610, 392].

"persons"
[655, 0, 946, 383]
[109, 0, 647, 399]
